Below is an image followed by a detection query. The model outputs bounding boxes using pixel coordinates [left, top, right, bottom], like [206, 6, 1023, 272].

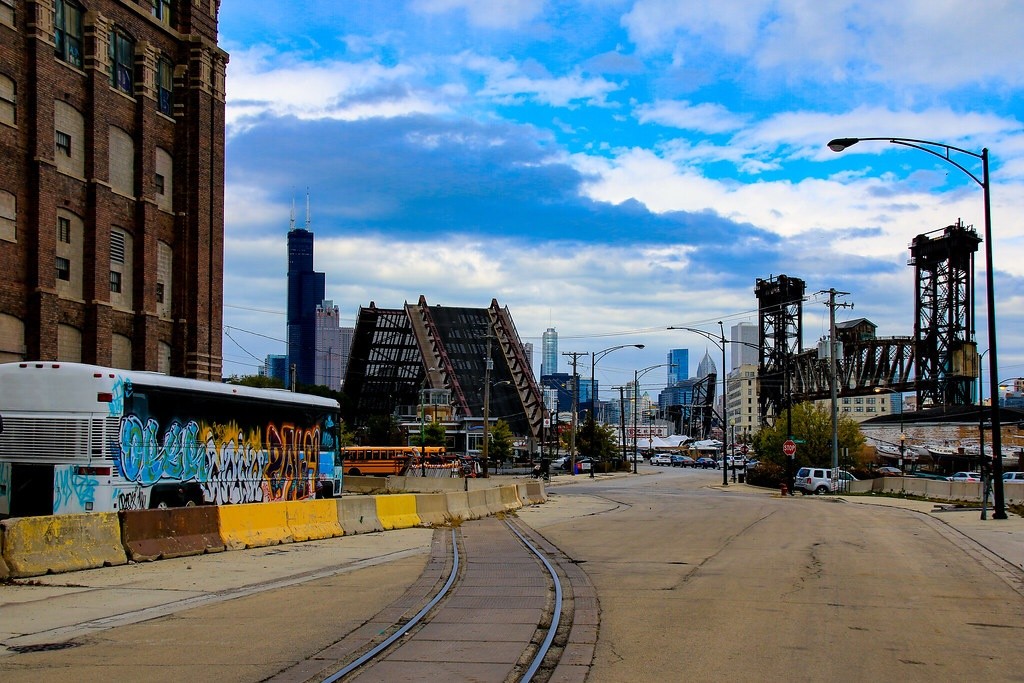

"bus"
[341, 446, 447, 476]
[0, 361, 340, 526]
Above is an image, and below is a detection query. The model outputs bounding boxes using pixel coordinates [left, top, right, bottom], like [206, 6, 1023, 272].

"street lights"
[730, 417, 737, 482]
[827, 135, 1009, 521]
[570, 408, 588, 476]
[667, 321, 729, 485]
[873, 386, 908, 477]
[590, 343, 646, 478]
[634, 363, 679, 474]
[419, 367, 436, 477]
[481, 380, 510, 480]
[717, 339, 796, 494]
[742, 422, 749, 476]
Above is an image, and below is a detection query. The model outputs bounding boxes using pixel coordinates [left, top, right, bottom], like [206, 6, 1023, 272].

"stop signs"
[783, 439, 796, 456]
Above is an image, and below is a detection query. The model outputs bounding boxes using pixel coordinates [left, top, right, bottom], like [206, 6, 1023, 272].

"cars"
[946, 471, 982, 483]
[876, 467, 903, 477]
[694, 457, 717, 469]
[626, 452, 644, 463]
[992, 471, 1024, 483]
[670, 455, 695, 468]
[746, 458, 763, 470]
[551, 455, 600, 472]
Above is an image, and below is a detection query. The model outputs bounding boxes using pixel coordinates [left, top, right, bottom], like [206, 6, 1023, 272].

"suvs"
[793, 467, 859, 496]
[650, 453, 671, 467]
[717, 455, 744, 470]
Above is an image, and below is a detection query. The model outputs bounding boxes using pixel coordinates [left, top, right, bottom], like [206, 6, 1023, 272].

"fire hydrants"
[779, 483, 788, 496]
